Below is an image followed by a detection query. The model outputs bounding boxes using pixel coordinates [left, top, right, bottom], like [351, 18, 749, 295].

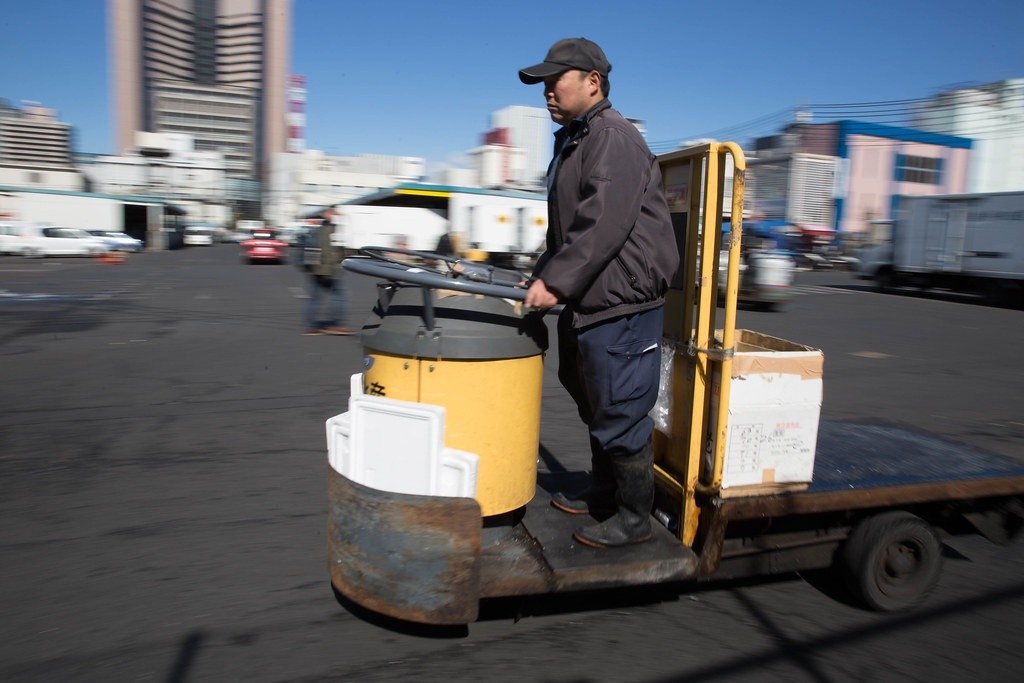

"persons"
[435, 225, 488, 264]
[515, 38, 679, 549]
[298, 208, 360, 336]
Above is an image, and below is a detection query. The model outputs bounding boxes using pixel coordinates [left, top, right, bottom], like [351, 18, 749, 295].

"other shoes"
[320, 326, 357, 334]
[300, 328, 322, 335]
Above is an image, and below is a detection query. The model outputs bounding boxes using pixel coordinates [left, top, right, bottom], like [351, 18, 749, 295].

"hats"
[519, 38, 609, 85]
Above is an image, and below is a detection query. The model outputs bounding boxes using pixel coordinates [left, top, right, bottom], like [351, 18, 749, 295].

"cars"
[0, 220, 872, 310]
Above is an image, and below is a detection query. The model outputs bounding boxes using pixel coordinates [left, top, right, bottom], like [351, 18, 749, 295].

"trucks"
[860, 190, 1024, 305]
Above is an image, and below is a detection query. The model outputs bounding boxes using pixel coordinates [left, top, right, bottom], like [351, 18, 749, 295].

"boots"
[551, 452, 614, 515]
[574, 451, 654, 549]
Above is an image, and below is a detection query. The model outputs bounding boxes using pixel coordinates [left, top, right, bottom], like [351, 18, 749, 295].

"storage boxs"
[691, 328, 823, 499]
